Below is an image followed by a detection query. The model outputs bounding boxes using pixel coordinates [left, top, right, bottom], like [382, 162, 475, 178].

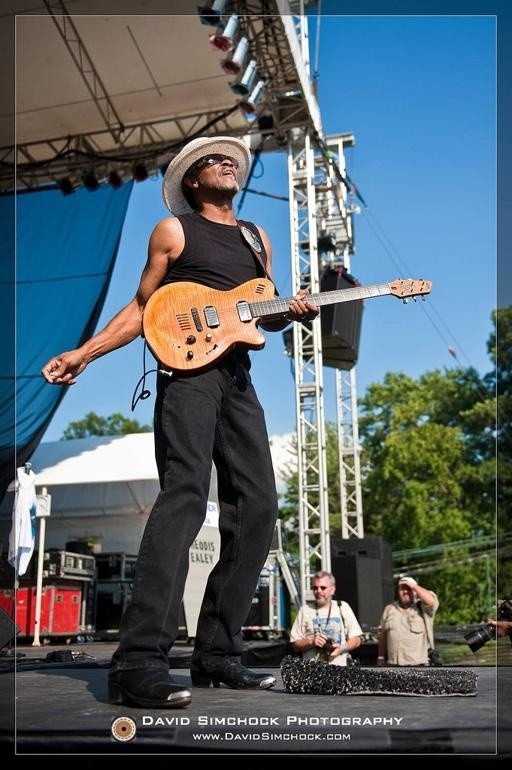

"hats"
[161, 136, 253, 215]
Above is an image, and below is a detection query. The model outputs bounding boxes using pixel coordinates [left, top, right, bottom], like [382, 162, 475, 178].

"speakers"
[328, 534, 394, 610]
[283, 263, 366, 371]
[333, 555, 383, 633]
[241, 571, 273, 633]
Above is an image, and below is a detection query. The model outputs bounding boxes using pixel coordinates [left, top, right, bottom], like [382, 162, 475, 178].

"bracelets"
[377, 656, 386, 660]
[341, 643, 349, 655]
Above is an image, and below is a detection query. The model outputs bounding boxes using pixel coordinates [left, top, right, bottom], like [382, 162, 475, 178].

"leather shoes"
[189, 660, 277, 691]
[108, 664, 192, 709]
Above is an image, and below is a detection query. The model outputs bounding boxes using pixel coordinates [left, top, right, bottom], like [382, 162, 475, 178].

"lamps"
[197, 1, 267, 115]
[54, 154, 159, 197]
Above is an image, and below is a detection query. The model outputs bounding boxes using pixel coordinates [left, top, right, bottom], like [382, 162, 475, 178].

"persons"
[376, 576, 441, 669]
[40, 135, 321, 709]
[289, 569, 365, 666]
[487, 598, 512, 642]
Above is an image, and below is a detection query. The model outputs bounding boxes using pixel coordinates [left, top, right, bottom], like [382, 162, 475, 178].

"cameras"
[318, 637, 334, 657]
[464, 598, 512, 652]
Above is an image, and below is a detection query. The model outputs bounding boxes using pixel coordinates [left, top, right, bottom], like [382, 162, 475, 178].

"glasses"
[189, 152, 239, 179]
[313, 586, 327, 590]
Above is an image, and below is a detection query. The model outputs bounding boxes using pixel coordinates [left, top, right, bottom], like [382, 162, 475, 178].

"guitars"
[141, 277, 431, 370]
